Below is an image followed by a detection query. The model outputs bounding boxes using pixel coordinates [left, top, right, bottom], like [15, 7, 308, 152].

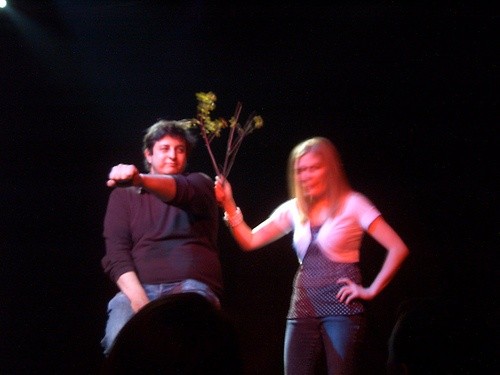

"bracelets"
[222, 206, 245, 228]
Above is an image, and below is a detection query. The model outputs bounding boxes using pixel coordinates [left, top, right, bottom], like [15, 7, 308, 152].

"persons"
[214, 137, 411, 375]
[100, 120, 227, 360]
